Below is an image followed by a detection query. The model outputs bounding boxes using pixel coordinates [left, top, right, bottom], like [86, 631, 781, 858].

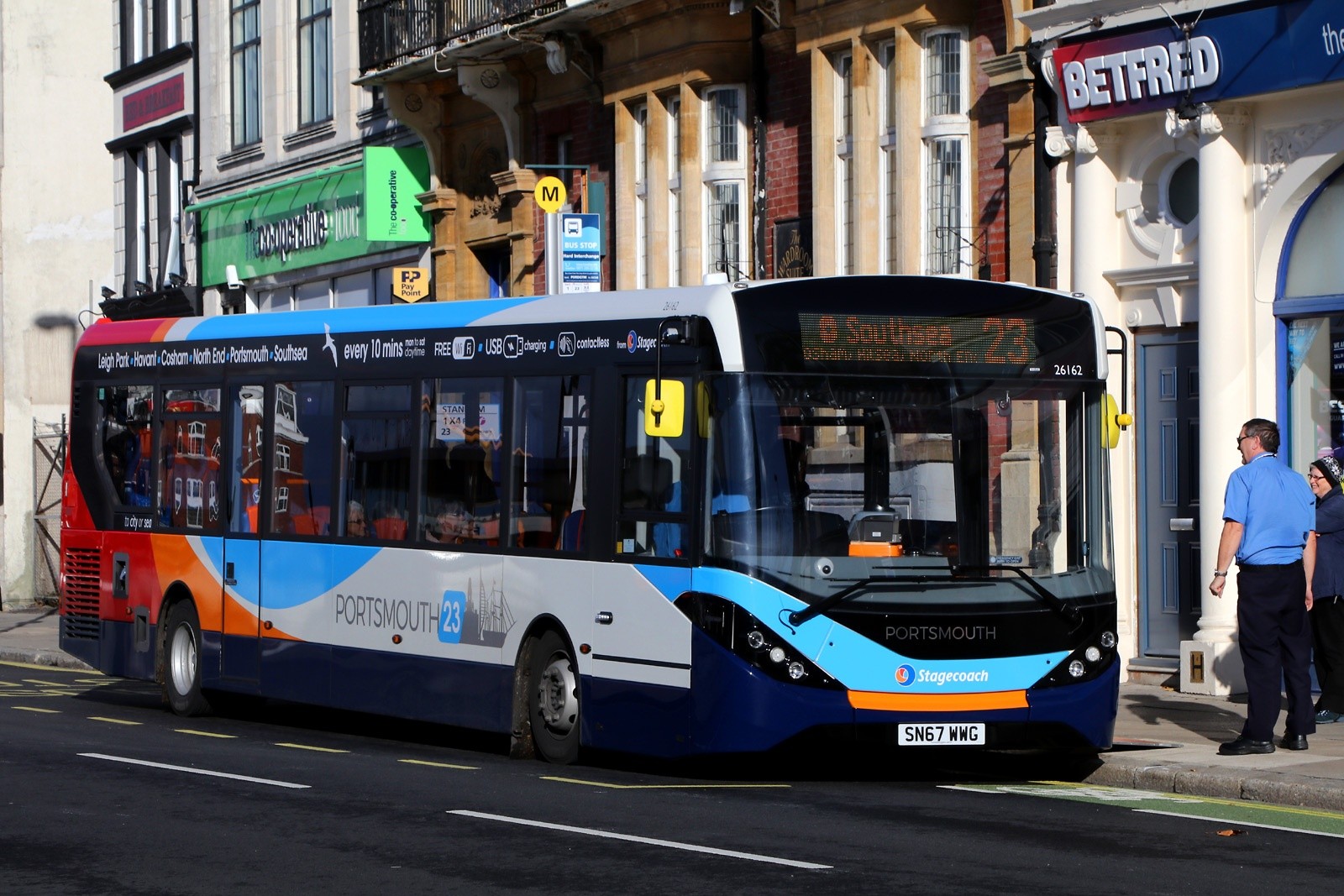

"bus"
[55, 274, 1131, 776]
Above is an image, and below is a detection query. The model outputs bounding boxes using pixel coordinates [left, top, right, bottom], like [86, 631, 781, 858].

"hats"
[1310, 456, 1344, 488]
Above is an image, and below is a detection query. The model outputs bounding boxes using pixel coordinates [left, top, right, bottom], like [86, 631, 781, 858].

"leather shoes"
[1283, 729, 1309, 749]
[1219, 733, 1276, 755]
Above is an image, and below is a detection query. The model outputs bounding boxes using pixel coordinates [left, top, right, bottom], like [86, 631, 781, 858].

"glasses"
[1308, 474, 1325, 480]
[1237, 435, 1254, 445]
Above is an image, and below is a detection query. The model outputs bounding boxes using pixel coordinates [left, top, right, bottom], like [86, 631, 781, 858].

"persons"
[425, 501, 465, 544]
[1209, 419, 1316, 755]
[1308, 456, 1344, 723]
[320, 500, 366, 537]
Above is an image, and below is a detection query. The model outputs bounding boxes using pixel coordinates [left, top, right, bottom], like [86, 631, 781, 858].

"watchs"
[1214, 568, 1227, 577]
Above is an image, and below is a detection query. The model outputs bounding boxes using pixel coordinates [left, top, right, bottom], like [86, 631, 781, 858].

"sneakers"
[1315, 710, 1344, 723]
